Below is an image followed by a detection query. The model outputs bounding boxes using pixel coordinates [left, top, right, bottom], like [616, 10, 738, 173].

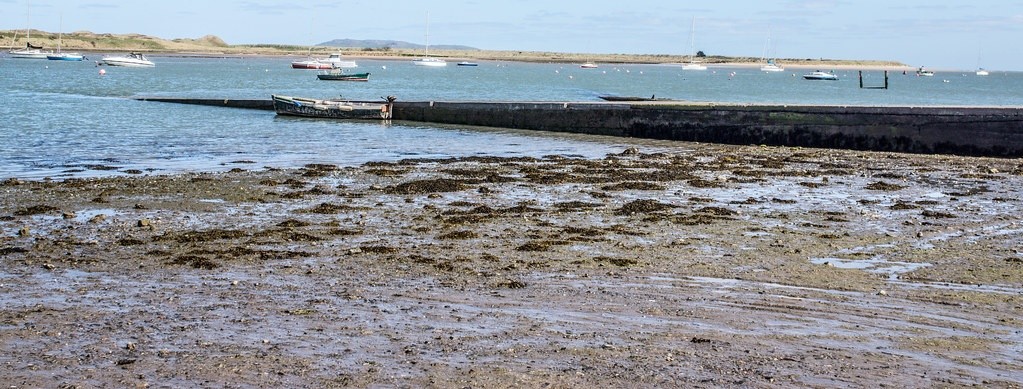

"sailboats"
[412, 9, 449, 67]
[681, 16, 708, 71]
[759, 33, 785, 71]
[46, 13, 89, 62]
[6, 0, 55, 59]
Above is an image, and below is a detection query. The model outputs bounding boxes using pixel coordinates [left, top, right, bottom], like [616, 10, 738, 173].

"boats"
[975, 67, 990, 78]
[270, 93, 396, 121]
[580, 59, 599, 69]
[318, 61, 371, 82]
[98, 51, 156, 69]
[916, 65, 935, 78]
[803, 69, 841, 82]
[290, 53, 358, 70]
[457, 61, 478, 67]
